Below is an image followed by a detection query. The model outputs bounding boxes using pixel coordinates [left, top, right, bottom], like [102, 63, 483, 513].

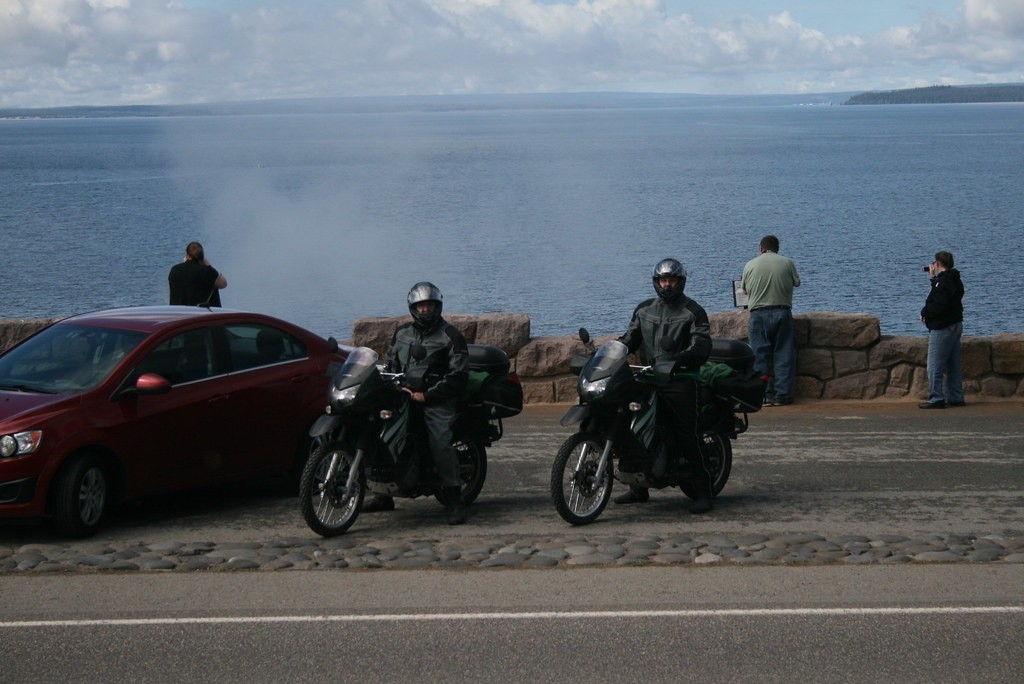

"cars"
[0, 304, 373, 542]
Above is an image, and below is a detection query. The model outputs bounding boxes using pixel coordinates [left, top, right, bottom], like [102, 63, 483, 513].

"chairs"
[108, 332, 144, 371]
[173, 336, 209, 385]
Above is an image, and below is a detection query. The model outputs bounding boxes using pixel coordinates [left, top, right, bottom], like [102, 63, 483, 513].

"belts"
[750, 305, 790, 312]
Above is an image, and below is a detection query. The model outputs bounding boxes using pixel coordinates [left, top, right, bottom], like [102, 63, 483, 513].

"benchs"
[230, 329, 284, 367]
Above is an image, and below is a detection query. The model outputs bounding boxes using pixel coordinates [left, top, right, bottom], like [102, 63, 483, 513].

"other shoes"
[761, 395, 773, 406]
[688, 496, 713, 513]
[945, 400, 966, 407]
[773, 397, 793, 406]
[613, 487, 650, 504]
[919, 400, 945, 409]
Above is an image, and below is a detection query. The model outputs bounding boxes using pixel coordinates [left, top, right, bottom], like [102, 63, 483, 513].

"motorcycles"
[298, 336, 524, 539]
[550, 327, 771, 526]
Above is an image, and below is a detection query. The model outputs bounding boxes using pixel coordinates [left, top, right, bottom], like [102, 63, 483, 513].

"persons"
[360, 281, 470, 525]
[740, 236, 801, 406]
[168, 243, 228, 307]
[919, 251, 966, 409]
[612, 258, 715, 513]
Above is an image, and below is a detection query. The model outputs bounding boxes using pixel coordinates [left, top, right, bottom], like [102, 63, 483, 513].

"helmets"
[407, 281, 443, 329]
[652, 258, 687, 303]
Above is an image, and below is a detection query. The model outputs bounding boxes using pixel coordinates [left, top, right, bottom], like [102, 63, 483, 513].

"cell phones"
[922, 266, 930, 273]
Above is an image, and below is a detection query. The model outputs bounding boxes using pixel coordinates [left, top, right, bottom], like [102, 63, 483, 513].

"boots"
[348, 495, 395, 512]
[442, 486, 467, 525]
